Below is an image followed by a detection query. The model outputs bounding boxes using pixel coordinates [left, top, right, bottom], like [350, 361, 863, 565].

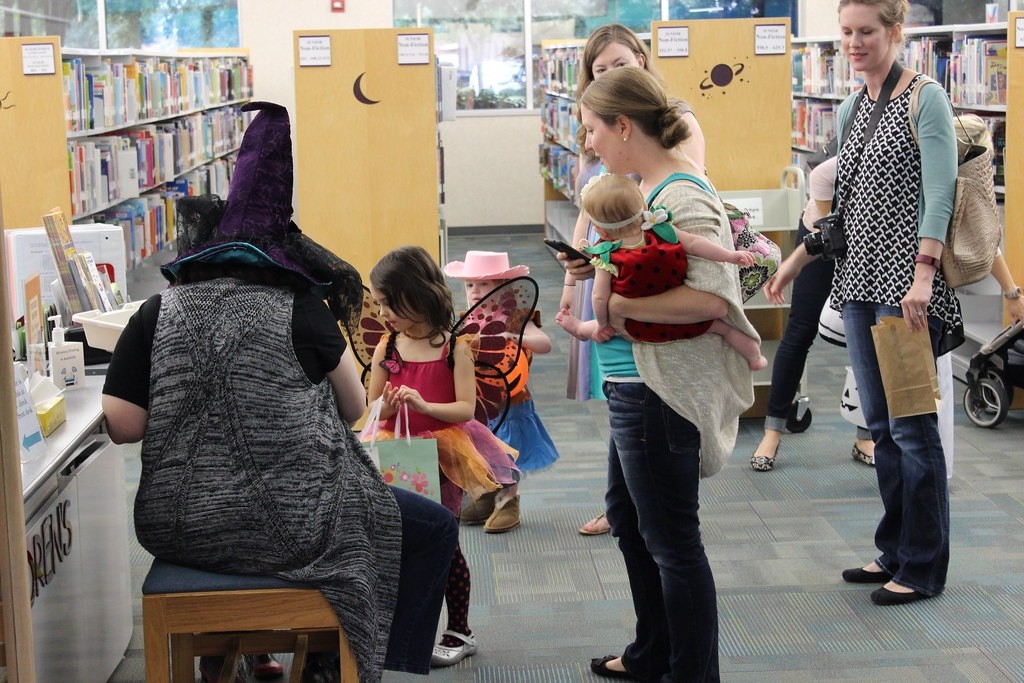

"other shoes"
[854, 444, 876, 467]
[203, 656, 245, 683]
[429, 629, 476, 666]
[590, 656, 632, 678]
[750, 437, 782, 471]
[249, 653, 284, 680]
[482, 492, 521, 532]
[842, 567, 895, 584]
[463, 488, 501, 525]
[871, 581, 927, 606]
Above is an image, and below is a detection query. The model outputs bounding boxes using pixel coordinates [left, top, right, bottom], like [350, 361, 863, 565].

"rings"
[917, 311, 923, 316]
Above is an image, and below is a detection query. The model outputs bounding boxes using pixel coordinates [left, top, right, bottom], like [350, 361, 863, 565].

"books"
[4, 208, 127, 335]
[895, 31, 1008, 237]
[60, 55, 255, 276]
[435, 54, 445, 282]
[539, 45, 590, 202]
[791, 41, 865, 186]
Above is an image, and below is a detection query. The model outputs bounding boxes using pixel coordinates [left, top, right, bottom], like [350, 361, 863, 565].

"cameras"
[802, 215, 848, 262]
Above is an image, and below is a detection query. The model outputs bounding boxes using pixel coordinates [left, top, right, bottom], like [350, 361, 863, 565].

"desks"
[18, 362, 132, 683]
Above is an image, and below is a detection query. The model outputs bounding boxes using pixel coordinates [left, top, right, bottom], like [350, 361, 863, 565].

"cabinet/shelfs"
[539, 16, 814, 422]
[640, 164, 807, 434]
[0, 36, 260, 281]
[788, 11, 1024, 411]
[286, 25, 446, 290]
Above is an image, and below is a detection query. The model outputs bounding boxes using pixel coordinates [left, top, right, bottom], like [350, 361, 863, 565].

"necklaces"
[402, 327, 444, 348]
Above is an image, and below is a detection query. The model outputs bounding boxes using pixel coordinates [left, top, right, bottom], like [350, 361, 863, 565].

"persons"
[443, 251, 552, 534]
[751, 155, 1024, 473]
[102, 194, 460, 683]
[750, 0, 1024, 606]
[551, 23, 769, 683]
[367, 246, 523, 665]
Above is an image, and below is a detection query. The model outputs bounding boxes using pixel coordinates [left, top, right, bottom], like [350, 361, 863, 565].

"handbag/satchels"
[359, 394, 442, 506]
[708, 178, 781, 304]
[870, 316, 941, 419]
[908, 80, 1001, 288]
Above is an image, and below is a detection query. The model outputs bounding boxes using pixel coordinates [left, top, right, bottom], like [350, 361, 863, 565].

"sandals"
[579, 511, 611, 535]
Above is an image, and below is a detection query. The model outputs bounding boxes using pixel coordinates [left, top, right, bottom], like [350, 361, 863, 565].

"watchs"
[1004, 286, 1022, 300]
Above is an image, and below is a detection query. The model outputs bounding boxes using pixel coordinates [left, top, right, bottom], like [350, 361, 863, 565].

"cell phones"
[543, 238, 592, 267]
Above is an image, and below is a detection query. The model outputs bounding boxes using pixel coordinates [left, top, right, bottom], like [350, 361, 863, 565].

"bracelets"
[914, 253, 940, 268]
[563, 284, 575, 286]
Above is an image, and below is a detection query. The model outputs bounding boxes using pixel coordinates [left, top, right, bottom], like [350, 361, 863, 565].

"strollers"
[962, 319, 1023, 431]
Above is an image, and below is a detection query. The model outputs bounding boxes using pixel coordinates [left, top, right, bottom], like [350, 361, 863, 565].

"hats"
[443, 251, 529, 281]
[163, 102, 363, 330]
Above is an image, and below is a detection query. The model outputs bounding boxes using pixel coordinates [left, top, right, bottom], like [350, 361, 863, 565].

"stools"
[141, 560, 366, 683]
